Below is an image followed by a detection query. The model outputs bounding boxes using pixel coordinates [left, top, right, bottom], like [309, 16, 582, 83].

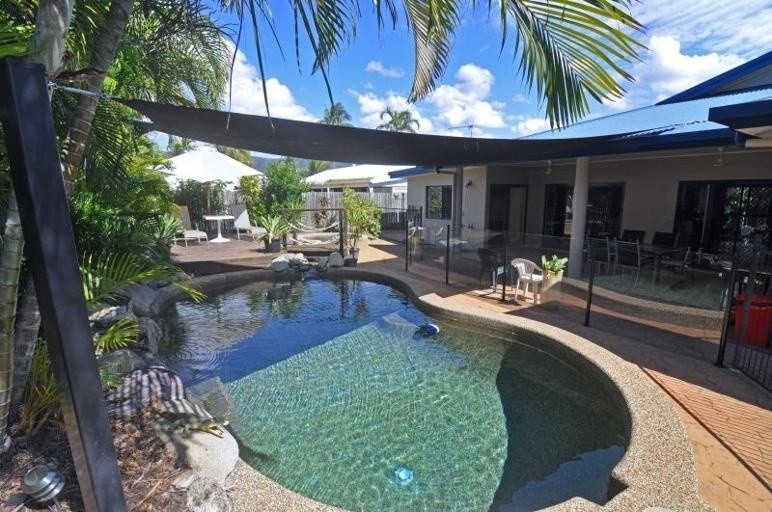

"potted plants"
[340, 186, 384, 261]
[540, 254, 568, 311]
[248, 213, 293, 254]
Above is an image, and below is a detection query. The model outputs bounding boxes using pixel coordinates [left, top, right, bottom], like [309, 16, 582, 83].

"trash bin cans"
[733, 293, 772, 352]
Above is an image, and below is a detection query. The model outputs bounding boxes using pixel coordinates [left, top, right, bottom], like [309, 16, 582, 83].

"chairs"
[231, 204, 252, 241]
[511, 257, 543, 306]
[409, 237, 424, 262]
[476, 247, 508, 292]
[583, 229, 696, 290]
[170, 204, 209, 248]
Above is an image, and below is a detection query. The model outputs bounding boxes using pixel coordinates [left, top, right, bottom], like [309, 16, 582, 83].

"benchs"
[282, 232, 340, 256]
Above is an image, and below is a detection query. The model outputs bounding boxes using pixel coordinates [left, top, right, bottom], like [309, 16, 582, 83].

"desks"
[202, 215, 238, 244]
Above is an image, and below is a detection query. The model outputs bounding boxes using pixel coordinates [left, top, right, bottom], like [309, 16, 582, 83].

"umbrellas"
[146, 147, 263, 214]
